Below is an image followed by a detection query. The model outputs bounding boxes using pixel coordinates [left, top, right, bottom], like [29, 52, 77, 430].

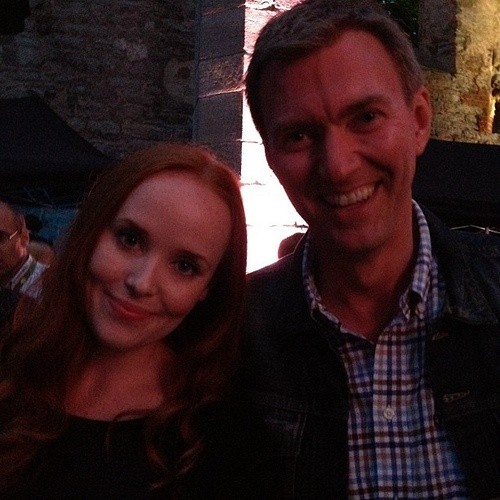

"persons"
[234, 0, 500, 499]
[0, 202, 52, 403]
[1, 144, 313, 500]
